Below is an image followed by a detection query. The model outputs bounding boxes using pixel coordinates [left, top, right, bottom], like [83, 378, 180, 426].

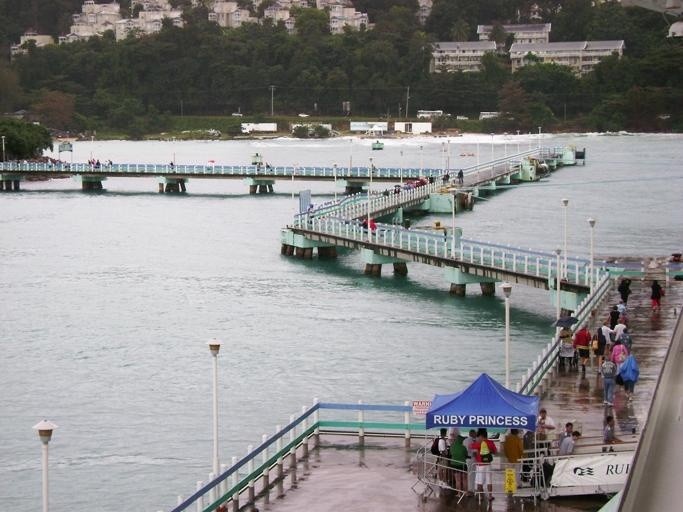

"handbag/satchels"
[619, 351, 626, 362]
[591, 331, 600, 351]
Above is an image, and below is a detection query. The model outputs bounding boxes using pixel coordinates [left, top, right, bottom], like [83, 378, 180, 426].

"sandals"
[454, 491, 495, 502]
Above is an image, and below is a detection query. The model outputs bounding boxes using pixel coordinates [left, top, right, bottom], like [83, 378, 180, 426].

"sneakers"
[438, 479, 450, 491]
[603, 399, 615, 407]
[559, 361, 586, 371]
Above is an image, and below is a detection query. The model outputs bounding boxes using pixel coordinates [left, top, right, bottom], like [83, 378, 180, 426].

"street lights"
[201, 334, 223, 507]
[494, 195, 598, 394]
[0, 131, 176, 166]
[31, 419, 58, 511]
[285, 123, 544, 262]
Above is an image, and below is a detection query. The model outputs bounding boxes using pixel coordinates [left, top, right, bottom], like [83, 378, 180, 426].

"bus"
[414, 108, 514, 121]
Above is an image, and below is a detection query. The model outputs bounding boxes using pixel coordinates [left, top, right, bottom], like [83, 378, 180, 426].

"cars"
[297, 112, 309, 117]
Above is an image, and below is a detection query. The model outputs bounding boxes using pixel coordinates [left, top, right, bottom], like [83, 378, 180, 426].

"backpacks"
[430, 435, 448, 456]
[479, 439, 493, 462]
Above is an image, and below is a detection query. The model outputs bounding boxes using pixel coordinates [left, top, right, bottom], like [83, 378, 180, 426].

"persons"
[618, 280, 633, 306]
[559, 300, 632, 371]
[603, 341, 639, 451]
[430, 408, 581, 500]
[651, 280, 663, 310]
[307, 204, 314, 224]
[17, 157, 67, 168]
[88, 158, 112, 168]
[360, 169, 464, 236]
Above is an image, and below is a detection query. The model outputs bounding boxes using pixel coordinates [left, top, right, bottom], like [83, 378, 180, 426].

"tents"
[425, 372, 540, 502]
